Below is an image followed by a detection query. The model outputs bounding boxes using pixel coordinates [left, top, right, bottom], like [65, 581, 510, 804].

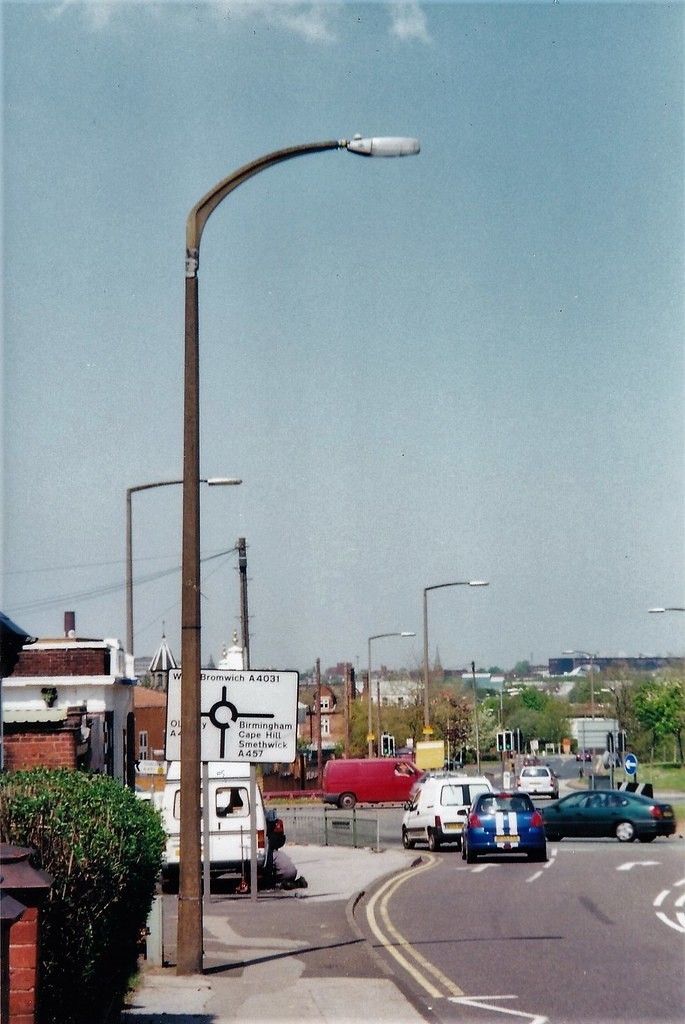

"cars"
[518, 764, 561, 799]
[534, 789, 677, 844]
[456, 792, 547, 864]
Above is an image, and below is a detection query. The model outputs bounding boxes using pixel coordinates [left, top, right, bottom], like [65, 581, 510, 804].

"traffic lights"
[504, 731, 511, 752]
[497, 732, 504, 752]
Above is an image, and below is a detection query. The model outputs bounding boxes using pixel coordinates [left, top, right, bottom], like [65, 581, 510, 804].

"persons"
[393, 763, 410, 778]
[262, 847, 308, 891]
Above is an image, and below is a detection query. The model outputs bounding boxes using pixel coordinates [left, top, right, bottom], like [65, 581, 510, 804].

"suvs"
[401, 774, 493, 851]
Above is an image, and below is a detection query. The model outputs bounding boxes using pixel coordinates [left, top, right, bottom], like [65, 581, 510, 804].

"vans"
[321, 756, 426, 809]
[159, 760, 274, 895]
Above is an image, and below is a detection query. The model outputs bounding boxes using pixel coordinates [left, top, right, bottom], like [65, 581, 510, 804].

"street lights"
[412, 579, 490, 732]
[176, 134, 422, 983]
[363, 627, 425, 754]
[117, 472, 243, 652]
